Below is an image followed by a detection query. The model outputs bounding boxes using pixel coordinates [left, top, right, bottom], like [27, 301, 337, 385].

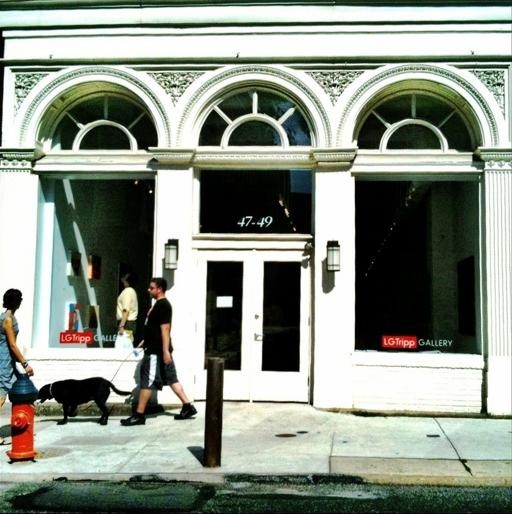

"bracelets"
[119, 325, 125, 327]
[22, 361, 28, 368]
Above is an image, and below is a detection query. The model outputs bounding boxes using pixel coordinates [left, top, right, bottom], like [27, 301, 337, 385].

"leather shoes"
[120, 414, 146, 426]
[174, 405, 197, 420]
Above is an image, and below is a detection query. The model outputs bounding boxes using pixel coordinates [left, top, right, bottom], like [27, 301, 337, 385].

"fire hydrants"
[5, 374, 38, 461]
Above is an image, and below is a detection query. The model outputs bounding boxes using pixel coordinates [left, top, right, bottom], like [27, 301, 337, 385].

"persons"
[0, 288, 34, 444]
[118, 278, 197, 423]
[116, 272, 140, 350]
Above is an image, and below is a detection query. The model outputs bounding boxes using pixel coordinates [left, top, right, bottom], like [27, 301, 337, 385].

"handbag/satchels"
[113, 329, 134, 348]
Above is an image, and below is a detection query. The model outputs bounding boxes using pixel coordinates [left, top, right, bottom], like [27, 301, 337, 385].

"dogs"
[36, 377, 132, 425]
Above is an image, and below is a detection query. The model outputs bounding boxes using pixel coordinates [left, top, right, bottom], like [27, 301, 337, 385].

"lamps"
[163, 239, 179, 270]
[325, 239, 341, 272]
